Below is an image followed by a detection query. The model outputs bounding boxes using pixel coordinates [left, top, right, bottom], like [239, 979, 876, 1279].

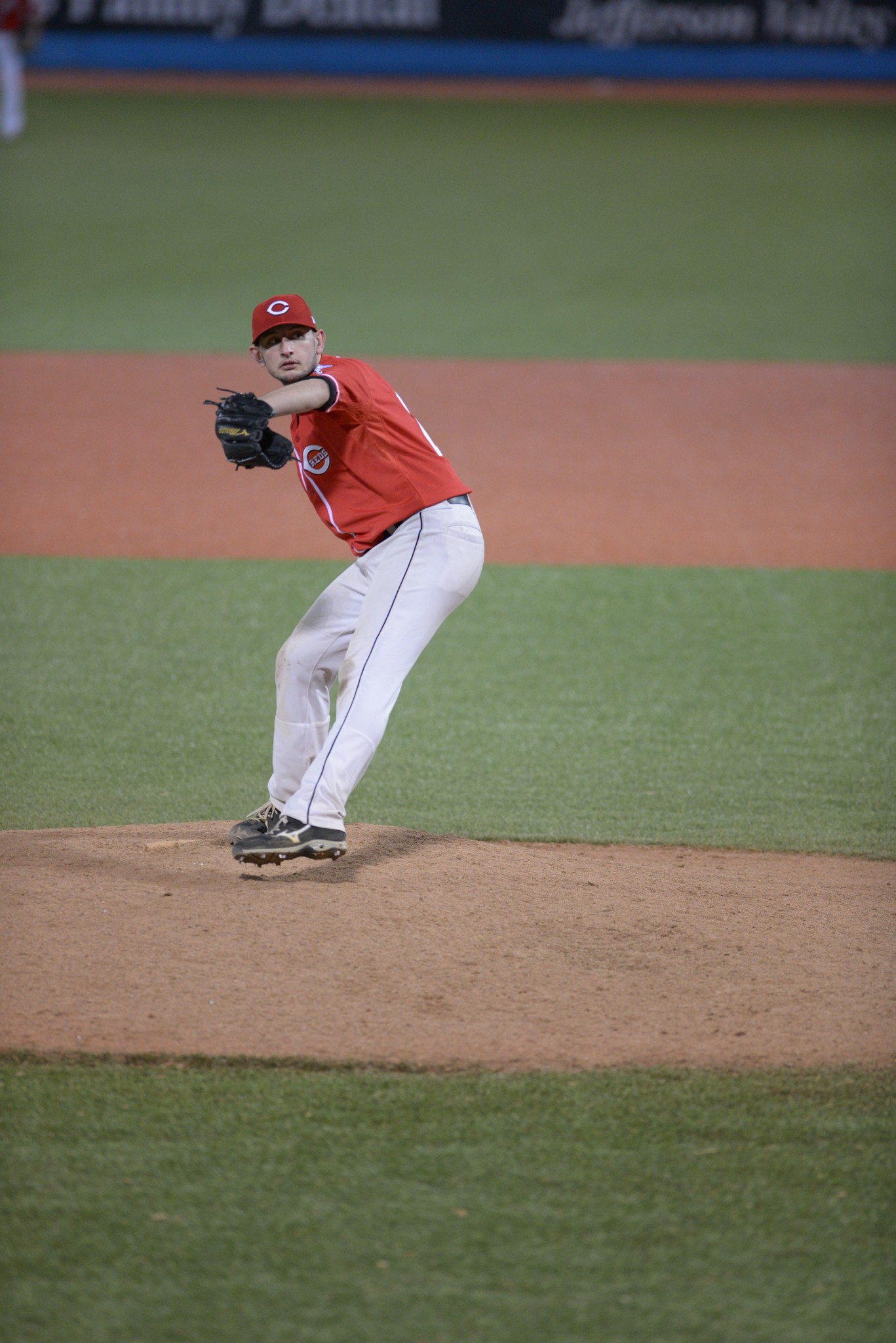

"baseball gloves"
[215, 393, 294, 471]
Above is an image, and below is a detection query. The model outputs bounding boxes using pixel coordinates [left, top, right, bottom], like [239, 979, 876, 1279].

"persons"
[0, 0, 32, 139]
[203, 294, 486, 868]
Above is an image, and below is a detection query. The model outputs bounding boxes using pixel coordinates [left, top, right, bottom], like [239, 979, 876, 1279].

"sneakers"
[232, 813, 347, 867]
[229, 798, 280, 846]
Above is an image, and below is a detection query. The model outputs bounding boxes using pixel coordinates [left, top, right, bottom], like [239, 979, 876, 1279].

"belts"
[382, 494, 470, 539]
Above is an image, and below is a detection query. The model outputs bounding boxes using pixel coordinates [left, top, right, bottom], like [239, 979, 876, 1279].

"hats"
[252, 294, 317, 344]
